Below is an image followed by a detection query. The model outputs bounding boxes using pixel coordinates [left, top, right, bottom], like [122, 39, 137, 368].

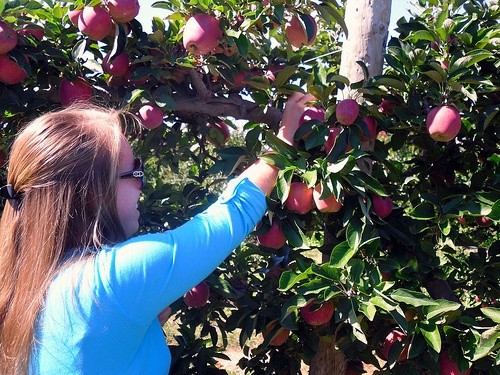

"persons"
[0, 92, 320, 375]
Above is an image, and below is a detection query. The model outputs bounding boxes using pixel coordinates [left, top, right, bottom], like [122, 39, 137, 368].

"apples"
[0, 0, 494, 375]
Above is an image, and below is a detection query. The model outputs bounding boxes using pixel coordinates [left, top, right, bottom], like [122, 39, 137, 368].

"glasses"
[110, 157, 144, 188]
